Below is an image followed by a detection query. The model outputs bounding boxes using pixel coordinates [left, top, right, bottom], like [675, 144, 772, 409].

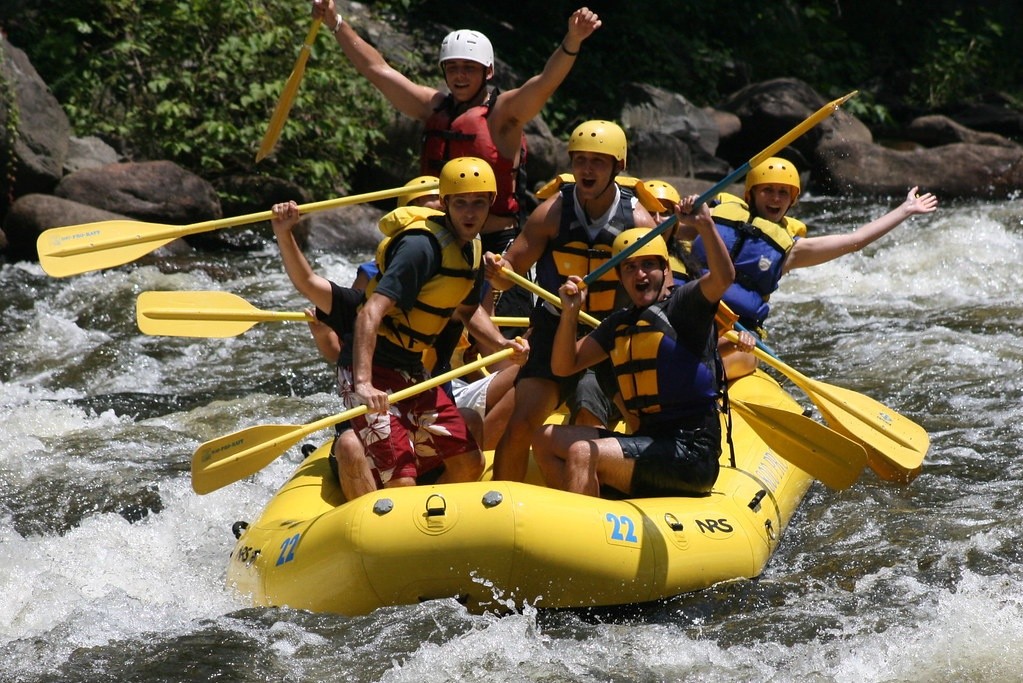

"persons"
[337, 156, 529, 488]
[272, 0, 938, 501]
[532, 193, 736, 501]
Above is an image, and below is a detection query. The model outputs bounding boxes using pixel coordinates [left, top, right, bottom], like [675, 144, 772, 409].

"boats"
[226, 240, 820, 616]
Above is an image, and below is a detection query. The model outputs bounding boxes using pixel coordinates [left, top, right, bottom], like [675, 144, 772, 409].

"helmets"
[439, 156, 497, 205]
[643, 180, 680, 234]
[438, 28, 495, 77]
[397, 176, 440, 211]
[744, 157, 800, 207]
[613, 228, 669, 270]
[568, 119, 627, 173]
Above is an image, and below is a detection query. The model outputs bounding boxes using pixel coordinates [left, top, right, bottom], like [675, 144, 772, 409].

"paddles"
[724, 330, 930, 469]
[135, 289, 530, 338]
[255, 14, 321, 164]
[489, 253, 868, 493]
[36, 182, 438, 279]
[737, 323, 921, 485]
[566, 88, 860, 295]
[191, 336, 524, 496]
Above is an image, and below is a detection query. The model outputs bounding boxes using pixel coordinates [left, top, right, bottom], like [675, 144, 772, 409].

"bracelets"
[330, 11, 345, 36]
